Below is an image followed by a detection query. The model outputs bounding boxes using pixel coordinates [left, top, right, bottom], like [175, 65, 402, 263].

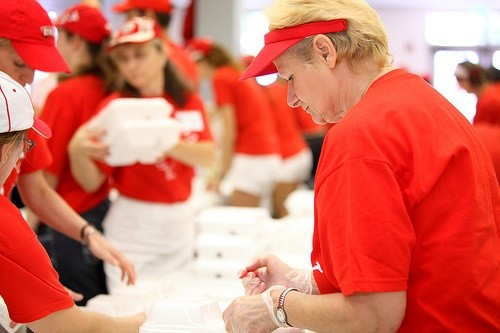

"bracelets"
[81, 222, 96, 247]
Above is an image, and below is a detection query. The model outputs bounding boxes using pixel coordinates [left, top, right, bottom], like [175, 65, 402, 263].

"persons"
[0, 0, 137, 303]
[66, 16, 216, 298]
[26, 4, 114, 308]
[454, 61, 499, 186]
[0, 71, 147, 333]
[222, 0, 499, 332]
[182, 38, 285, 208]
[110, 0, 202, 94]
[239, 55, 314, 220]
[484, 66, 499, 83]
[295, 106, 328, 192]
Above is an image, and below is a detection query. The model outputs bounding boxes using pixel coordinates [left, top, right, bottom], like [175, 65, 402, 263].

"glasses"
[24, 136, 34, 153]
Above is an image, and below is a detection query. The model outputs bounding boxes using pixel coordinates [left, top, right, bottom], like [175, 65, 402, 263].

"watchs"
[275, 288, 300, 328]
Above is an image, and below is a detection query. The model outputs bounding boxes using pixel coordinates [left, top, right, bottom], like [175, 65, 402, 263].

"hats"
[0, 0, 72, 75]
[1, 70, 53, 139]
[237, 19, 346, 82]
[183, 40, 213, 63]
[102, 17, 164, 55]
[52, 5, 112, 44]
[111, 0, 173, 14]
